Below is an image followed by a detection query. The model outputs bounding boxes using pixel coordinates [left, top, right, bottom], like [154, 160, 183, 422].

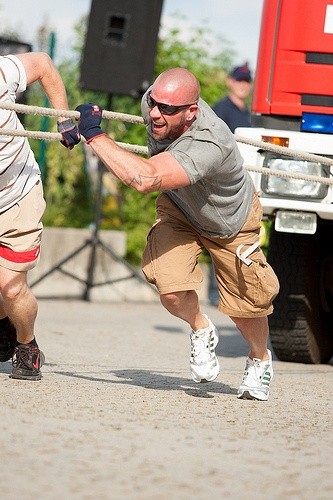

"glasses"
[146, 90, 193, 116]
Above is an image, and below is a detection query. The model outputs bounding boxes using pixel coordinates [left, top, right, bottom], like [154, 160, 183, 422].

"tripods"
[30, 94, 160, 302]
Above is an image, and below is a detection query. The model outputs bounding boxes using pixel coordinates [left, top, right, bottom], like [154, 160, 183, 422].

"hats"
[229, 67, 252, 83]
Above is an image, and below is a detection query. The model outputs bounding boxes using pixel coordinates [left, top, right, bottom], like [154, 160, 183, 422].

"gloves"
[74, 103, 107, 144]
[55, 119, 81, 149]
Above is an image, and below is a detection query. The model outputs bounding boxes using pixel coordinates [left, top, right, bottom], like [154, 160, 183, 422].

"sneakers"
[9, 342, 45, 381]
[237, 349, 273, 400]
[189, 314, 219, 383]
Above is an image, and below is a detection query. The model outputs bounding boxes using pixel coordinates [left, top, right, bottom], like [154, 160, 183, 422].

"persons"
[0, 52, 80, 379]
[211, 65, 251, 134]
[74, 67, 279, 400]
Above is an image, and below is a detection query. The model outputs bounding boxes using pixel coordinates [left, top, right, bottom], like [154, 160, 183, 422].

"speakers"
[79, 0, 164, 98]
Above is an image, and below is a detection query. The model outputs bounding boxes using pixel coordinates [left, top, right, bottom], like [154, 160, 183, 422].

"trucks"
[230, 1, 333, 364]
